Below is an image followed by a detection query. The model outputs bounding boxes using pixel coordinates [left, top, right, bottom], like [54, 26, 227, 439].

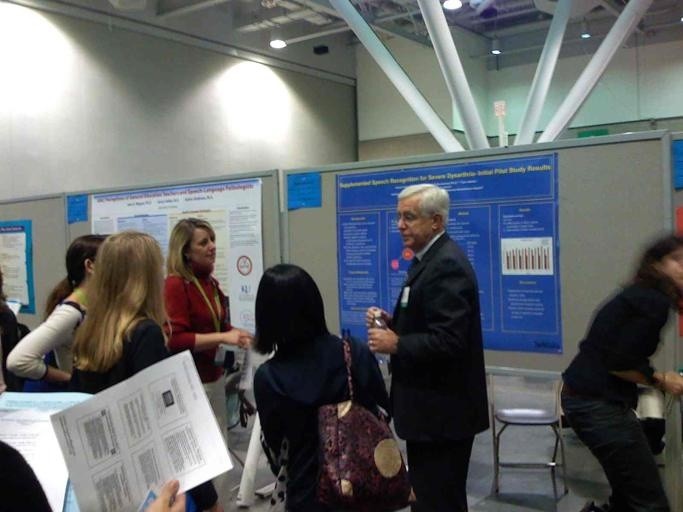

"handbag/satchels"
[225, 388, 243, 428]
[21, 352, 67, 393]
[317, 399, 417, 512]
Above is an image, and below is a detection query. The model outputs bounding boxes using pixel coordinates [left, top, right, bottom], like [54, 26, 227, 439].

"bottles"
[370, 311, 390, 363]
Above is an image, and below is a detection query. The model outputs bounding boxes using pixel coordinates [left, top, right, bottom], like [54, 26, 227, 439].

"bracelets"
[654, 371, 668, 388]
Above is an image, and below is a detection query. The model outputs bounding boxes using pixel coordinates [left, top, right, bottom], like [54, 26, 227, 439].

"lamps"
[578, 17, 589, 40]
[490, 0, 502, 55]
[268, 27, 287, 50]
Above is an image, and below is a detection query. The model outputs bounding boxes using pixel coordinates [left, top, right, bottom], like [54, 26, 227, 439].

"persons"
[252, 261, 394, 511]
[365, 183, 490, 512]
[161, 217, 254, 463]
[0, 233, 189, 511]
[562, 233, 682, 510]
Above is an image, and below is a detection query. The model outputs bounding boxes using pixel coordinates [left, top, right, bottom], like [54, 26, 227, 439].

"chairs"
[483, 366, 570, 503]
